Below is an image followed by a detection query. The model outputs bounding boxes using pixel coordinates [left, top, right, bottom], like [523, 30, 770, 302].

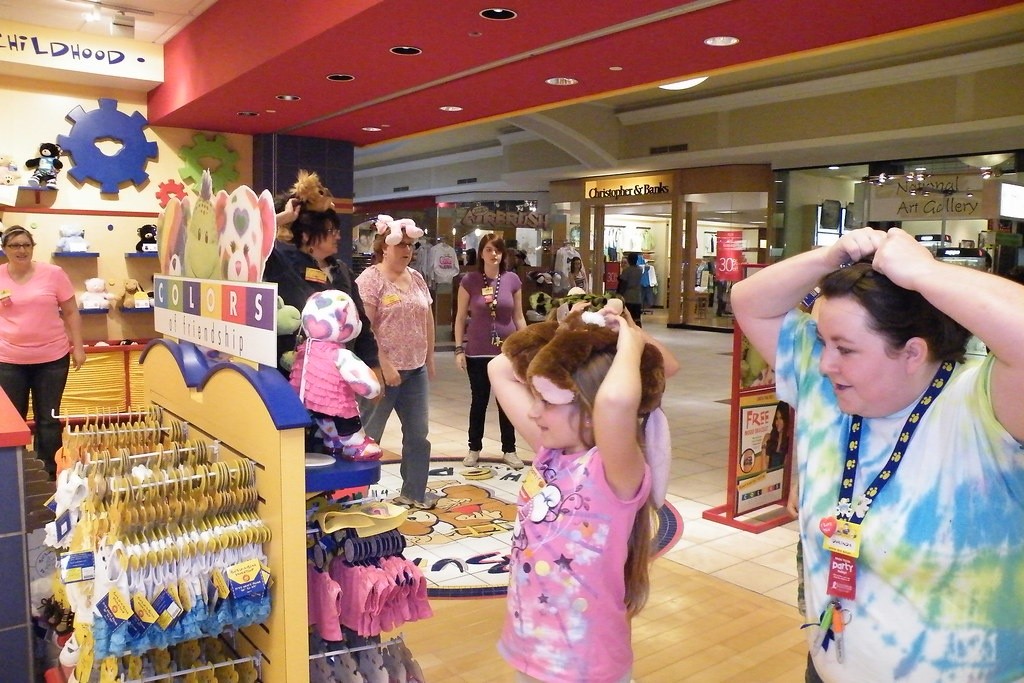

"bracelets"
[743, 457, 753, 465]
[455, 346, 465, 355]
[762, 444, 768, 449]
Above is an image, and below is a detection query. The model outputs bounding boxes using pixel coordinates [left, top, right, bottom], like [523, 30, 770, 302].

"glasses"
[4, 242, 33, 249]
[397, 241, 415, 249]
[327, 228, 341, 235]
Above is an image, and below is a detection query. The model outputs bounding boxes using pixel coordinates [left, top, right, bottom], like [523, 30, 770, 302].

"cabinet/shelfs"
[43, 335, 435, 683]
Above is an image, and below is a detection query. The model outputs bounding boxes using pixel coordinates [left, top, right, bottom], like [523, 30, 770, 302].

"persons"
[759, 402, 789, 472]
[465, 248, 476, 265]
[511, 253, 531, 272]
[0, 225, 87, 481]
[570, 257, 586, 291]
[454, 233, 529, 469]
[488, 306, 653, 683]
[263, 198, 435, 511]
[280, 286, 383, 461]
[613, 253, 644, 328]
[730, 227, 1024, 683]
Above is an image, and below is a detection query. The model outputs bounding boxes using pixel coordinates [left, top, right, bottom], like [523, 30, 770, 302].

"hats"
[529, 270, 564, 288]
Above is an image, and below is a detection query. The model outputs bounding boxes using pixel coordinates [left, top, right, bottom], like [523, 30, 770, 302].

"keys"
[491, 330, 498, 345]
[810, 602, 844, 664]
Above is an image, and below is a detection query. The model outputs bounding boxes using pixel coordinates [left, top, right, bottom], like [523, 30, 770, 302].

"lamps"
[861, 167, 1004, 198]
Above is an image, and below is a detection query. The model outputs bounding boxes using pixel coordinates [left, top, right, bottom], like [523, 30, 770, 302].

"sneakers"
[399, 492, 435, 509]
[503, 452, 524, 468]
[463, 448, 479, 466]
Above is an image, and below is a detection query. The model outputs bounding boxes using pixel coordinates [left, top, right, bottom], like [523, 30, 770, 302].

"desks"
[681, 293, 709, 319]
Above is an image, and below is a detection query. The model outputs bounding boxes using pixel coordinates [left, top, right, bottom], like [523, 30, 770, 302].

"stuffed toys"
[57, 223, 90, 253]
[272, 166, 335, 240]
[135, 224, 158, 252]
[375, 214, 424, 246]
[82, 278, 143, 309]
[0, 157, 23, 185]
[502, 301, 667, 417]
[27, 142, 64, 189]
[278, 296, 304, 339]
